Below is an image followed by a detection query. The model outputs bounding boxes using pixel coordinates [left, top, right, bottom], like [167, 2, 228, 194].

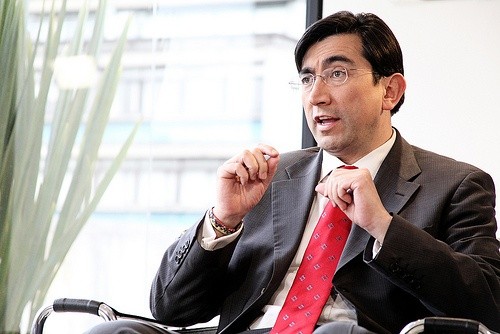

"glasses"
[288, 66, 383, 93]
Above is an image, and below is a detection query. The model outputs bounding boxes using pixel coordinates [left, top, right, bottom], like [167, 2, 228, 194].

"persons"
[85, 10, 500, 334]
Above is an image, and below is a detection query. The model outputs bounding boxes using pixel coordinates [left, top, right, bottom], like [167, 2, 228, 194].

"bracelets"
[208, 207, 239, 235]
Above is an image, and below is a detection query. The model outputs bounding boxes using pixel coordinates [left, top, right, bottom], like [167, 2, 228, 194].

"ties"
[263, 164, 366, 334]
